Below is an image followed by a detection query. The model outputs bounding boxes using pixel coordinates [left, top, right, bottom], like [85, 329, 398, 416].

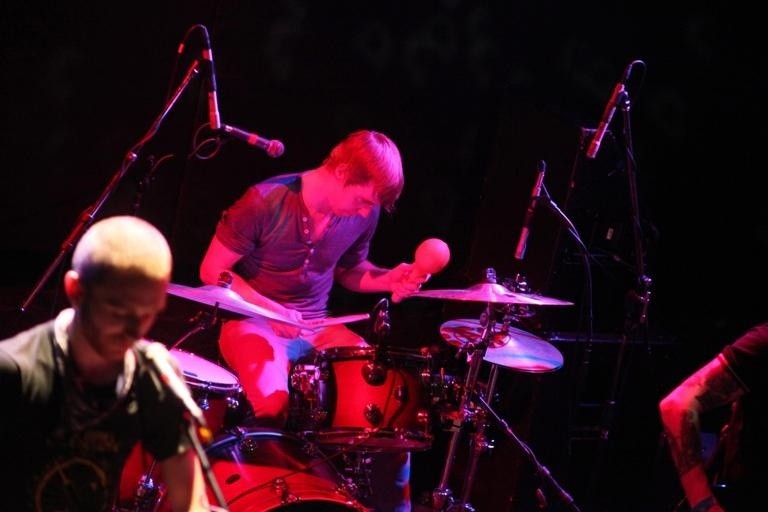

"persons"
[659, 325, 768, 512]
[200, 129, 431, 512]
[0, 215, 210, 512]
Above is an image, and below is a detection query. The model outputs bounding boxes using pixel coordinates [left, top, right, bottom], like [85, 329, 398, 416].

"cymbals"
[440, 319, 563, 374]
[402, 284, 573, 307]
[164, 283, 315, 332]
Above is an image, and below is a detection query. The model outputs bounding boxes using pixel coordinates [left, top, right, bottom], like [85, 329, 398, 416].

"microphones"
[217, 121, 285, 158]
[143, 341, 207, 428]
[511, 169, 547, 260]
[584, 81, 624, 160]
[390, 237, 451, 307]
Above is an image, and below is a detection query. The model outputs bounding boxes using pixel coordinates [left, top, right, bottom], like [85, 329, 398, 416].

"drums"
[288, 347, 434, 452]
[150, 428, 371, 512]
[117, 349, 243, 499]
[434, 373, 490, 433]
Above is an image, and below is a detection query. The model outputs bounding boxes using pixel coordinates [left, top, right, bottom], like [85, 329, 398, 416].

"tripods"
[459, 303, 518, 512]
[431, 302, 497, 512]
